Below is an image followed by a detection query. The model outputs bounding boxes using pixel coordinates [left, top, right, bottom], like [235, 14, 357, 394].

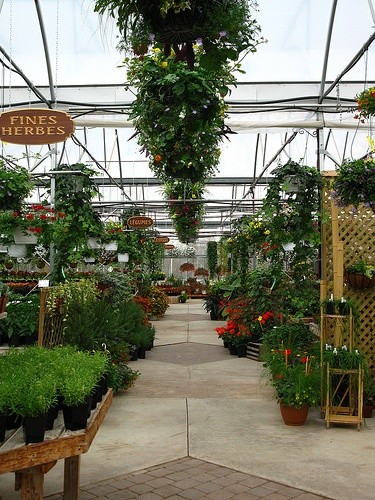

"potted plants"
[44, 162, 104, 239]
[0, 345, 113, 446]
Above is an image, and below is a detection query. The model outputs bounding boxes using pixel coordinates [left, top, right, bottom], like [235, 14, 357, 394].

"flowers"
[0, 0, 375, 403]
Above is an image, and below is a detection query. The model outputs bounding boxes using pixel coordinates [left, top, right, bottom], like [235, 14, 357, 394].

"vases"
[224, 341, 227, 348]
[279, 401, 309, 425]
[348, 272, 374, 290]
